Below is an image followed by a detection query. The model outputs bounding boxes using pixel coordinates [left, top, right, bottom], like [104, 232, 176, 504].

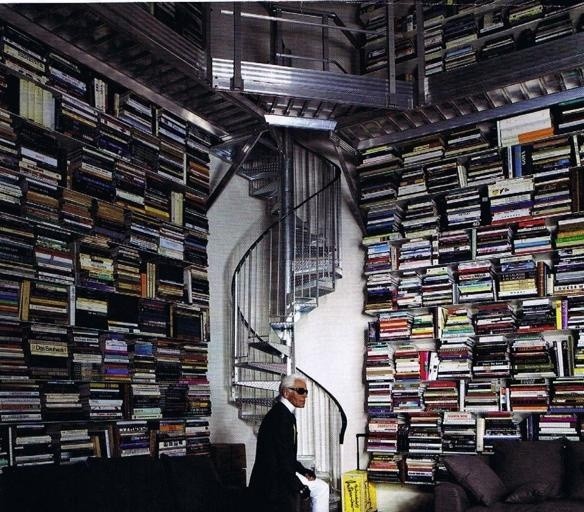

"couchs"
[433, 439, 583, 511]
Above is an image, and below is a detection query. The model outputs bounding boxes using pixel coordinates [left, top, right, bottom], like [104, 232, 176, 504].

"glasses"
[289, 387, 309, 395]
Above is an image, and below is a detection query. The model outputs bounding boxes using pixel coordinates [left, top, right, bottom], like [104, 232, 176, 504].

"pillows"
[441, 438, 569, 506]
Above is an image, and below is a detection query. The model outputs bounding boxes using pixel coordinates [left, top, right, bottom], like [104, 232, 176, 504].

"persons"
[245, 374, 316, 510]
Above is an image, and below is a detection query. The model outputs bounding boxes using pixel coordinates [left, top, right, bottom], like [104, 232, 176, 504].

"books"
[357, 1, 583, 80]
[0, 36, 213, 467]
[356, 100, 584, 482]
